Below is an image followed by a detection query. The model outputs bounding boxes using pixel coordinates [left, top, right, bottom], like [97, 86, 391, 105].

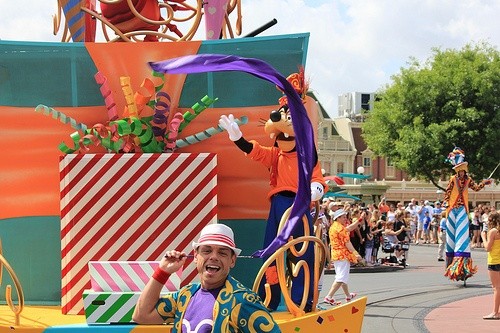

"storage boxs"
[82, 289, 141, 326]
[87, 260, 184, 293]
[58, 153, 217, 315]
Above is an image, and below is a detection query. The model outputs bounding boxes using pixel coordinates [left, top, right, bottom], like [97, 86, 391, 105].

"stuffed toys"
[218, 103, 328, 313]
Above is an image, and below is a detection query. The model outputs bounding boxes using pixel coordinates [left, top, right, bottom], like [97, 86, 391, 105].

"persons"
[444, 162, 492, 243]
[132, 223, 282, 333]
[480, 214, 500, 319]
[310, 197, 496, 265]
[324, 209, 366, 304]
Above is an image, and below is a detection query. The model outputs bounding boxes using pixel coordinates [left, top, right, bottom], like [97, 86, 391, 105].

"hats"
[320, 205, 326, 209]
[425, 200, 429, 205]
[425, 211, 428, 214]
[436, 201, 440, 205]
[192, 224, 242, 256]
[322, 198, 329, 203]
[333, 209, 348, 220]
[329, 202, 337, 209]
[409, 203, 413, 207]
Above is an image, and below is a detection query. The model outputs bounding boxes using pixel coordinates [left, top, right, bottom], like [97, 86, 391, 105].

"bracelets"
[358, 218, 362, 222]
[152, 268, 170, 284]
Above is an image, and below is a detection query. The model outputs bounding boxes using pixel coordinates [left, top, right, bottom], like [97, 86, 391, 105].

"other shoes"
[324, 297, 336, 305]
[367, 263, 374, 266]
[482, 312, 500, 319]
[372, 263, 380, 266]
[345, 293, 356, 302]
[410, 238, 437, 244]
[438, 258, 444, 261]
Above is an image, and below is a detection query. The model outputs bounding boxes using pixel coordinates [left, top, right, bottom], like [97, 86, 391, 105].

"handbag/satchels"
[379, 235, 384, 243]
